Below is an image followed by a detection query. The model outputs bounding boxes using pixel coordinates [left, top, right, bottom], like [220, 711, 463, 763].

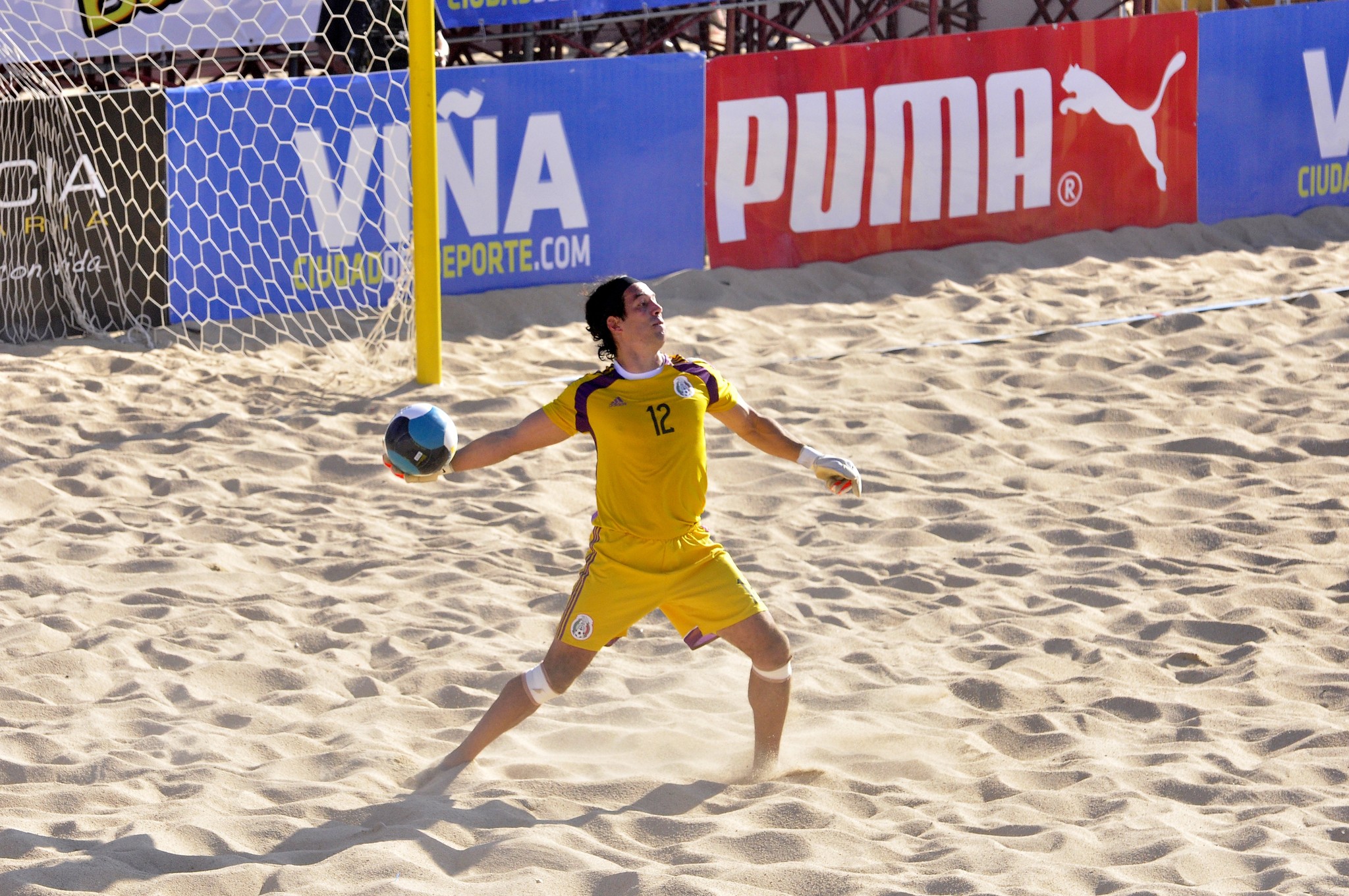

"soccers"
[383, 403, 458, 478]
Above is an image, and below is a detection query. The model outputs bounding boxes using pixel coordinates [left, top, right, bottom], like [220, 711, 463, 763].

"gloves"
[795, 444, 863, 497]
[384, 452, 454, 483]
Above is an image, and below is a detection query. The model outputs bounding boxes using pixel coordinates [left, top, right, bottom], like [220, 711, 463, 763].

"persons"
[380, 276, 861, 791]
